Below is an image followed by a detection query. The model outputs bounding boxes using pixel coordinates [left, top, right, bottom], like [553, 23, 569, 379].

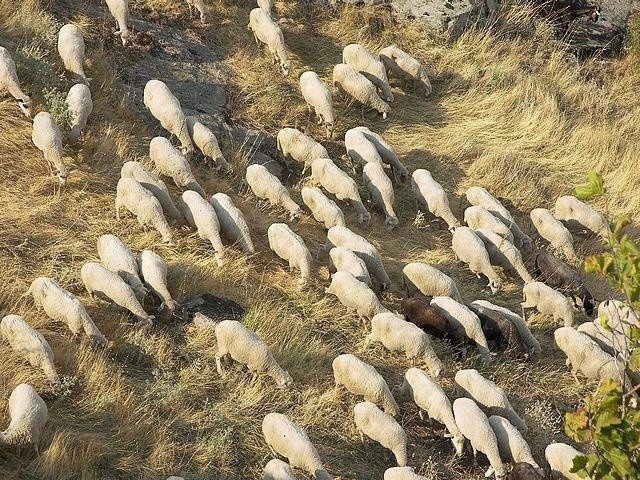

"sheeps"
[0, 0, 640, 479]
